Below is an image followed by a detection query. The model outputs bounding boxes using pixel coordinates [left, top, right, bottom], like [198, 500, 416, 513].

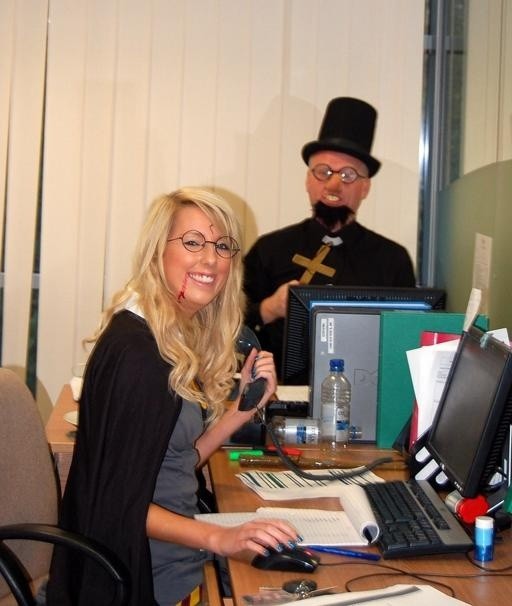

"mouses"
[252, 546, 320, 573]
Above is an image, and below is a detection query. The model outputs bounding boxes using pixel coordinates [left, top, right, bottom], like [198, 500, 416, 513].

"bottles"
[319, 357, 353, 467]
[268, 414, 363, 448]
[474, 516, 495, 562]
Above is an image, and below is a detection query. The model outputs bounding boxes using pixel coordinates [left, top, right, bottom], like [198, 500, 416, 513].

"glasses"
[168, 229, 240, 259]
[311, 163, 365, 184]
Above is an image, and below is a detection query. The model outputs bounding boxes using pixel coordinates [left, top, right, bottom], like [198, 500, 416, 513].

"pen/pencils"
[308, 545, 381, 560]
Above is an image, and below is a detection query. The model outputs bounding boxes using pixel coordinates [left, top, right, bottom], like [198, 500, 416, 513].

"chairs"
[1, 363, 132, 605]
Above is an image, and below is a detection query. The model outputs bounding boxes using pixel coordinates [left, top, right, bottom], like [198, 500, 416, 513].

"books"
[279, 582, 470, 606]
[374, 307, 511, 449]
[190, 484, 381, 550]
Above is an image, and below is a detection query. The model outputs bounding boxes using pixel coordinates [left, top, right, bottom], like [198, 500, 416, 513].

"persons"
[243, 151, 419, 418]
[49, 183, 306, 606]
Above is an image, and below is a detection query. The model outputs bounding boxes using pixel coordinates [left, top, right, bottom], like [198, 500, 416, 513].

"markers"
[229, 449, 280, 460]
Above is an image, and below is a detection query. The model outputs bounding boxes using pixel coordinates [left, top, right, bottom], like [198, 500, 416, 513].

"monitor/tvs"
[425, 325, 512, 536]
[281, 285, 448, 387]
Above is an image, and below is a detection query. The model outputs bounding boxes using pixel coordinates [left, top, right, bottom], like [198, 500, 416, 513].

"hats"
[301, 97, 381, 178]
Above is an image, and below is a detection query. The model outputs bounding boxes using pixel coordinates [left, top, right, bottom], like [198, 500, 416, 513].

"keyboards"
[359, 479, 473, 560]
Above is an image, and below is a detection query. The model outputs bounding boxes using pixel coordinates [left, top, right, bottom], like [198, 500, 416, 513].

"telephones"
[238, 327, 265, 410]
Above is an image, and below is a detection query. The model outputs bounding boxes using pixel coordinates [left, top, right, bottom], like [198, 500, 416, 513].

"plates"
[64, 410, 80, 429]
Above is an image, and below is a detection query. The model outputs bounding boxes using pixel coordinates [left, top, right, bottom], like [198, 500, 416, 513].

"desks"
[199, 444, 512, 606]
[45, 381, 83, 494]
